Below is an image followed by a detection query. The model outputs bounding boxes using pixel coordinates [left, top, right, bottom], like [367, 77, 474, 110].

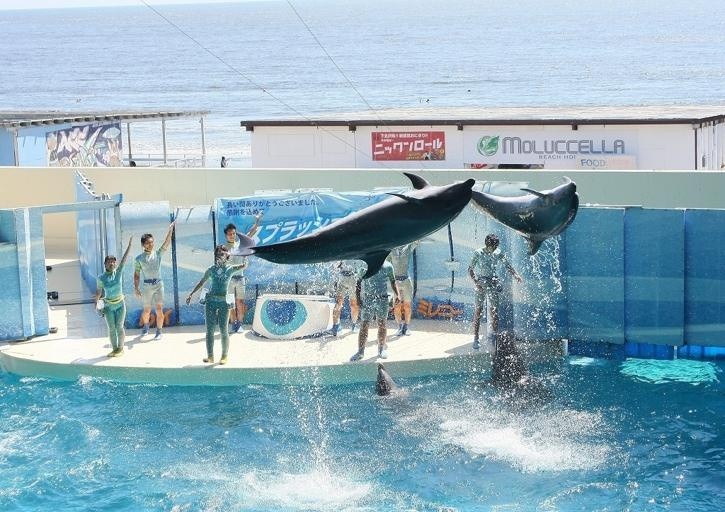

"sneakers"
[107, 349, 123, 357]
[203, 354, 227, 364]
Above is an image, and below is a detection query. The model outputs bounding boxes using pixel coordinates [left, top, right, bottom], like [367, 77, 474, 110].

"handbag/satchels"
[95, 299, 105, 317]
[198, 289, 208, 304]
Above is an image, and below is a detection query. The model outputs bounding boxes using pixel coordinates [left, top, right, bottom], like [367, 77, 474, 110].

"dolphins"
[217, 172, 476, 282]
[373, 361, 399, 397]
[401, 172, 580, 257]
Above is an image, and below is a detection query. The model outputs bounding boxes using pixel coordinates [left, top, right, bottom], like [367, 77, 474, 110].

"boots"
[351, 345, 387, 360]
[228, 320, 244, 334]
[141, 323, 162, 340]
[472, 333, 496, 349]
[396, 322, 410, 336]
[327, 324, 341, 336]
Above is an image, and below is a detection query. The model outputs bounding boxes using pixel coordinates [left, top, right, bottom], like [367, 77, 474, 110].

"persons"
[218, 210, 262, 333]
[329, 261, 360, 339]
[94, 235, 133, 357]
[184, 246, 246, 365]
[347, 263, 402, 363]
[383, 242, 421, 337]
[467, 235, 523, 349]
[132, 216, 178, 338]
